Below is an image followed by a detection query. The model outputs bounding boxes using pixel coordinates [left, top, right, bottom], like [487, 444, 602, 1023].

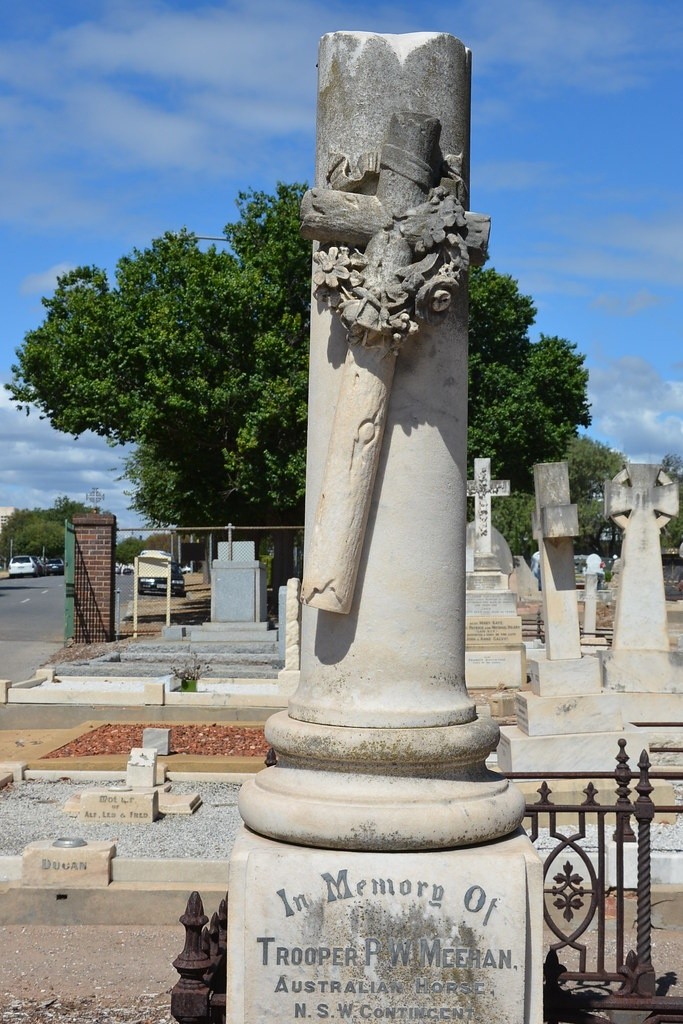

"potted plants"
[171, 650, 214, 692]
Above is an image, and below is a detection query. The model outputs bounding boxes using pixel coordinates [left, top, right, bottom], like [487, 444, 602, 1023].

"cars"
[44, 558, 64, 575]
[138, 550, 184, 595]
[8, 555, 44, 578]
